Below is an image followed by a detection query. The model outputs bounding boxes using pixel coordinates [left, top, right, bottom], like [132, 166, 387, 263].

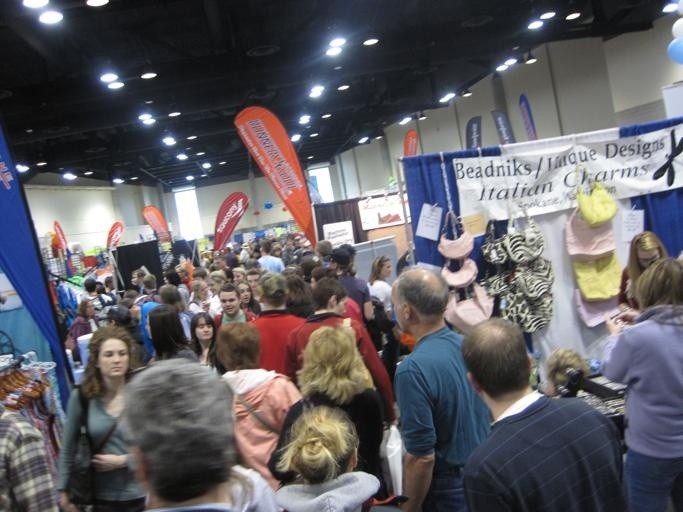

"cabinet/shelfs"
[352, 234, 398, 284]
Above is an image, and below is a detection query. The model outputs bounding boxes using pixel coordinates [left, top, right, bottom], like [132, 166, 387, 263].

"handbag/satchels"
[67, 432, 95, 504]
[437, 218, 553, 333]
[565, 185, 625, 328]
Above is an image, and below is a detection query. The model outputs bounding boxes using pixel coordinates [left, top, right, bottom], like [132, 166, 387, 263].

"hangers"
[0, 351, 56, 417]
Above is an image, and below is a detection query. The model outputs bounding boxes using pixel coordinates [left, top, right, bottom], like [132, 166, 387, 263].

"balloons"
[667, 37, 683, 64]
[672, 18, 683, 39]
[677, 0, 683, 16]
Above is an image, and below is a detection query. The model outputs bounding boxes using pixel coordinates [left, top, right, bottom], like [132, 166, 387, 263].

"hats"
[232, 268, 246, 276]
[98, 305, 131, 323]
[331, 248, 351, 266]
[303, 255, 322, 270]
[272, 242, 282, 251]
[241, 243, 250, 249]
[209, 271, 227, 281]
[257, 273, 289, 298]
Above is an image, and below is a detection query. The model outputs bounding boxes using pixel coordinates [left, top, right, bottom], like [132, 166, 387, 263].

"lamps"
[358, 41, 537, 144]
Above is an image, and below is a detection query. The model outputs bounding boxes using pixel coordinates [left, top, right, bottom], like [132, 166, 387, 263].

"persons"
[2, 232, 682, 510]
